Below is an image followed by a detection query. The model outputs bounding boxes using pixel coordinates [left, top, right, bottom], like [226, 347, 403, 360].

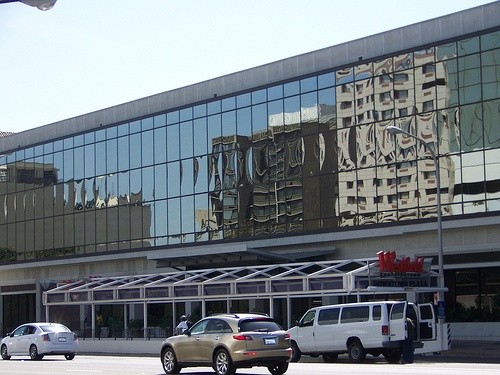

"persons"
[176, 315, 192, 335]
[400, 318, 415, 364]
[96, 311, 104, 327]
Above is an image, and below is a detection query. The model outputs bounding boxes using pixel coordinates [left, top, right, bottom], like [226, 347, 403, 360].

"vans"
[286, 300, 439, 362]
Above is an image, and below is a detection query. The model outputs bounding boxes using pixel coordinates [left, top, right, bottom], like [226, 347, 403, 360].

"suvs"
[159, 312, 294, 375]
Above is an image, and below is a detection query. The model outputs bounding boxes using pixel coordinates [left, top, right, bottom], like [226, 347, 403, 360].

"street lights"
[387, 125, 446, 314]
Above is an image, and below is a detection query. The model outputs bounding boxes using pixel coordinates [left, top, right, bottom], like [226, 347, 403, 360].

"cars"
[1, 321, 78, 363]
